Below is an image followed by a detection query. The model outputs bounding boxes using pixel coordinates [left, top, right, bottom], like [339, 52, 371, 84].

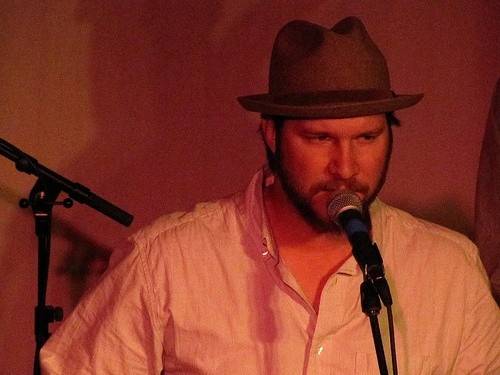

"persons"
[39, 15, 500, 375]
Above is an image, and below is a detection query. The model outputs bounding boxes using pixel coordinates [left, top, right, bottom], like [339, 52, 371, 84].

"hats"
[236, 16, 424, 119]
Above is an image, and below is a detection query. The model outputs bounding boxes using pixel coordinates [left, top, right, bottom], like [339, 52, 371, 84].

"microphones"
[327, 191, 393, 306]
